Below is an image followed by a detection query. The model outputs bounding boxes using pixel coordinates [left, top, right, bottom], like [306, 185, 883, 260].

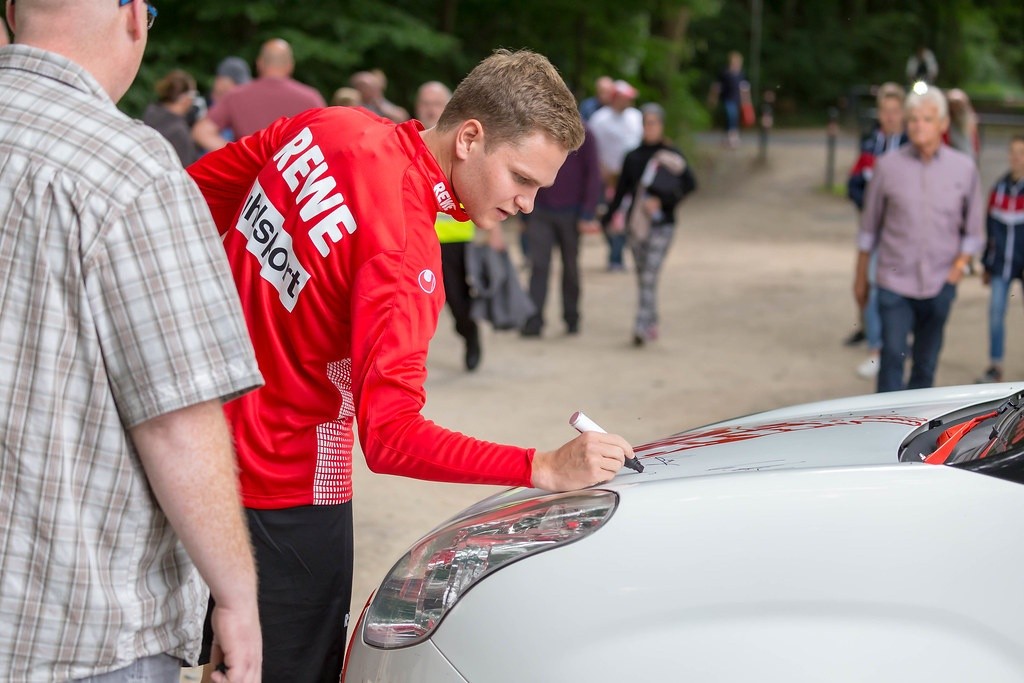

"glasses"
[120, 0, 157, 29]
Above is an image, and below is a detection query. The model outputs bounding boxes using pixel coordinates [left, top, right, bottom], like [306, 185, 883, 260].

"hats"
[610, 80, 638, 98]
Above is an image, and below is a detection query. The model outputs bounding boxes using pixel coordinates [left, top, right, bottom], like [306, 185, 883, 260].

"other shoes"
[635, 324, 649, 345]
[978, 368, 1004, 384]
[466, 338, 482, 370]
[567, 322, 578, 335]
[844, 330, 868, 346]
[522, 318, 541, 335]
[858, 357, 881, 377]
[608, 261, 625, 273]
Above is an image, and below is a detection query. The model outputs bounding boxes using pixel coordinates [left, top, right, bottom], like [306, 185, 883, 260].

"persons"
[519, 129, 601, 333]
[843, 87, 912, 376]
[187, 49, 635, 683]
[947, 90, 980, 276]
[337, 74, 407, 120]
[711, 54, 749, 146]
[587, 81, 643, 270]
[976, 136, 1024, 383]
[466, 224, 531, 328]
[597, 104, 695, 344]
[143, 70, 198, 167]
[0, 0, 263, 683]
[417, 81, 482, 368]
[183, 42, 326, 155]
[853, 87, 987, 395]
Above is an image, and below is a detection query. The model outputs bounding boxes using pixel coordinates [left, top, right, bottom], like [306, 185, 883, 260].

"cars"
[341, 375, 1023, 683]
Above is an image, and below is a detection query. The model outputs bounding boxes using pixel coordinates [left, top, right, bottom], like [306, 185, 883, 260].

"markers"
[569, 409, 645, 473]
